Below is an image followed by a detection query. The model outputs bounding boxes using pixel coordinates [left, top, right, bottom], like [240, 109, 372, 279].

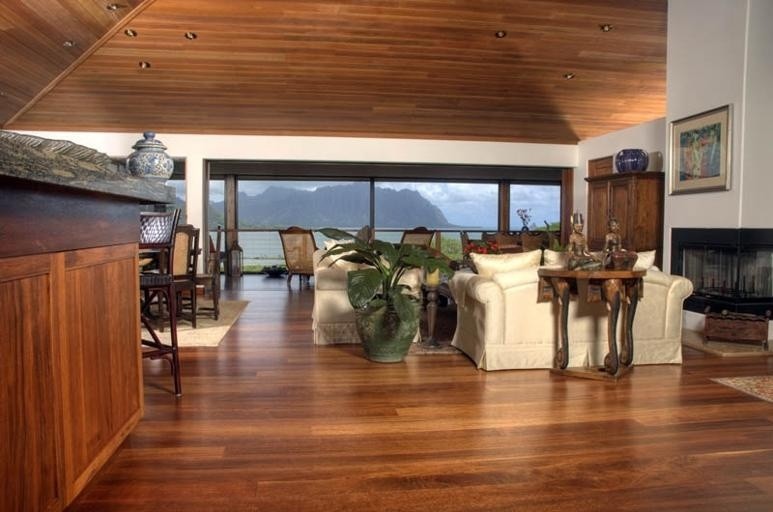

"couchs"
[308, 249, 423, 350]
[449, 250, 695, 374]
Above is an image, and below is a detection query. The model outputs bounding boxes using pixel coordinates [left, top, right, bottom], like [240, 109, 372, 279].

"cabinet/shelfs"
[704, 312, 768, 349]
[584, 172, 665, 271]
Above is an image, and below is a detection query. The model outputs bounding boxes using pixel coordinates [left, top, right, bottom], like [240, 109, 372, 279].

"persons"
[604, 218, 627, 270]
[568, 214, 602, 270]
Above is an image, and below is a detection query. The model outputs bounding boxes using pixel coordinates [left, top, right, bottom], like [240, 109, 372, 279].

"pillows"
[469, 250, 543, 278]
[543, 248, 657, 271]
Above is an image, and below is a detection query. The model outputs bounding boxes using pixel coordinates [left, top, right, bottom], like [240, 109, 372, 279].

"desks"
[533, 265, 648, 380]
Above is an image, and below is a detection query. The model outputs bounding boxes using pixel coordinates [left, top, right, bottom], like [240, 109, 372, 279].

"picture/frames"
[667, 102, 734, 195]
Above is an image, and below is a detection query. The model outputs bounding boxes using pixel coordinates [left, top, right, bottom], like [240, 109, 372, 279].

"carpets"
[707, 375, 773, 402]
[681, 327, 770, 358]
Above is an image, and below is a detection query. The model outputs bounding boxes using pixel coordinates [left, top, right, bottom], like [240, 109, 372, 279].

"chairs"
[277, 225, 317, 289]
[139, 208, 220, 398]
[520, 231, 545, 251]
[459, 231, 476, 268]
[496, 234, 520, 253]
[401, 226, 433, 248]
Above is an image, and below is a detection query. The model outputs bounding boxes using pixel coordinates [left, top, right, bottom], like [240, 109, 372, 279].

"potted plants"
[314, 225, 455, 364]
